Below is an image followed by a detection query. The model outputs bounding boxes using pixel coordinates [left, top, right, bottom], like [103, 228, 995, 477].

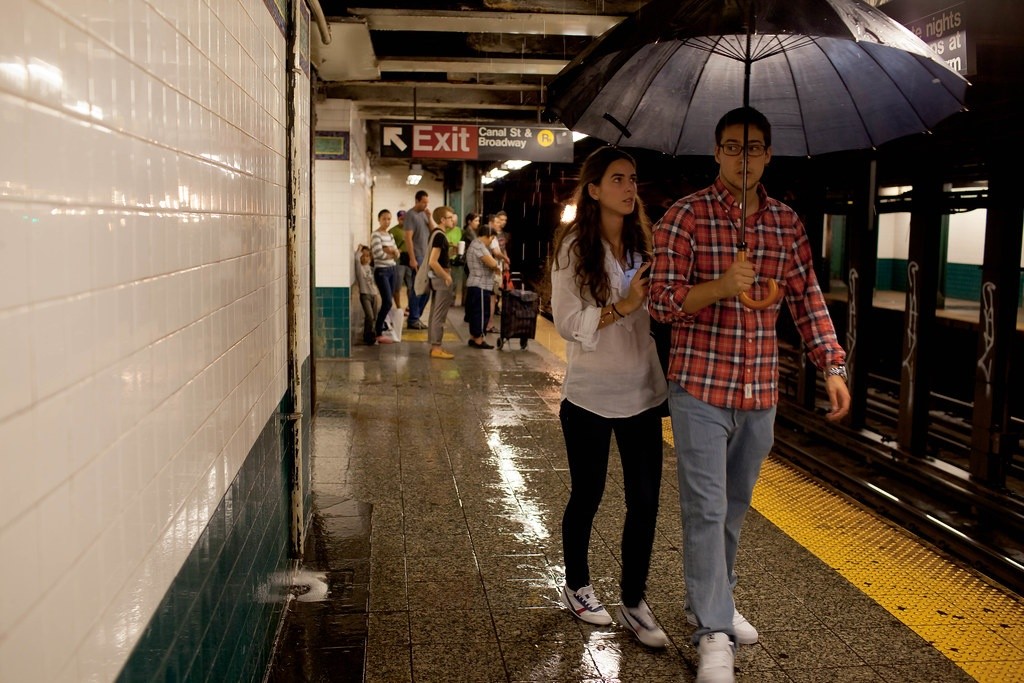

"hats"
[397, 210, 407, 217]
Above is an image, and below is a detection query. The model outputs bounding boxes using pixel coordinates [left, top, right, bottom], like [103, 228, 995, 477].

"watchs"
[823, 366, 848, 382]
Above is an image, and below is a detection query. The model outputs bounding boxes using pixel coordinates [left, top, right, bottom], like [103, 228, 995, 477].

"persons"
[550, 145, 670, 647]
[648, 106, 850, 683]
[355, 190, 514, 359]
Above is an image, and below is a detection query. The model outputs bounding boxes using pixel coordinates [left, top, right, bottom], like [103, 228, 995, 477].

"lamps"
[405, 86, 590, 186]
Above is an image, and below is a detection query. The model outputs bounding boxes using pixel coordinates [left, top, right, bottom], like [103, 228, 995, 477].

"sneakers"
[561, 582, 613, 625]
[694, 631, 736, 683]
[616, 600, 669, 648]
[686, 606, 759, 644]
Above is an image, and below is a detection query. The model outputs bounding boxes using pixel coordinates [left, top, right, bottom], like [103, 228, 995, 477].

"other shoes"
[429, 347, 455, 359]
[468, 339, 495, 349]
[406, 319, 428, 330]
[494, 310, 502, 315]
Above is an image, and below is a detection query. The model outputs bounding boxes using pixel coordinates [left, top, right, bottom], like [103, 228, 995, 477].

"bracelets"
[600, 302, 624, 324]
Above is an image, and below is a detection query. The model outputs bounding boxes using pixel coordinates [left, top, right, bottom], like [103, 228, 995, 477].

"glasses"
[444, 216, 453, 219]
[718, 143, 767, 157]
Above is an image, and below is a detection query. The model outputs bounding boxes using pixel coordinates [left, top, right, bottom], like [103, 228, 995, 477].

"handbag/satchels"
[377, 305, 404, 343]
[413, 231, 448, 296]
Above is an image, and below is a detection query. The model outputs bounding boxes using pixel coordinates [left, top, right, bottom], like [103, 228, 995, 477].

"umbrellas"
[542, 1, 973, 309]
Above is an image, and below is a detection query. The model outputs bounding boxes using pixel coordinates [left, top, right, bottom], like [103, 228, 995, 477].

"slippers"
[486, 325, 500, 334]
